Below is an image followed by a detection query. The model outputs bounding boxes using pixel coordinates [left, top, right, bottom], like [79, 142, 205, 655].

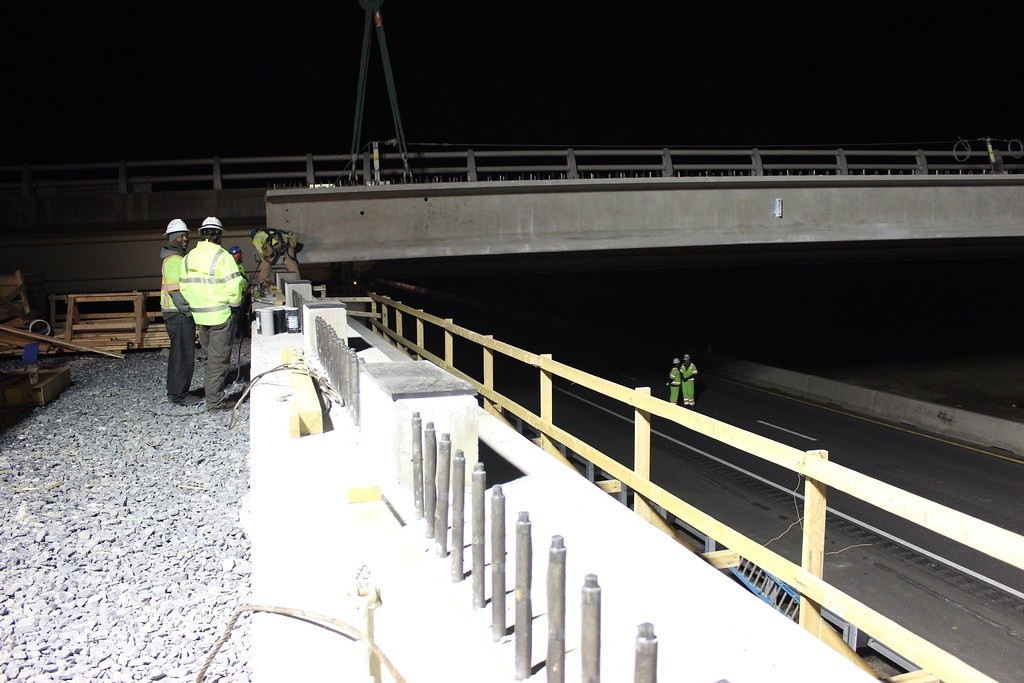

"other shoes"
[175, 392, 200, 406]
[210, 400, 236, 413]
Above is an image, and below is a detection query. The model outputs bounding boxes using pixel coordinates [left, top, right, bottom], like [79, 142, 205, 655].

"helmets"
[673, 358, 680, 364]
[163, 219, 191, 237]
[683, 354, 690, 360]
[198, 217, 225, 237]
[251, 228, 258, 240]
[228, 245, 242, 255]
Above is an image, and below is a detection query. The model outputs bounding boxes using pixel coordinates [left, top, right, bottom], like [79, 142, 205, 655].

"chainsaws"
[254, 278, 267, 302]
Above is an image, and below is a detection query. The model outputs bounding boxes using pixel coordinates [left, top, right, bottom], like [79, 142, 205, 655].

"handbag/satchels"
[286, 232, 298, 249]
[262, 241, 274, 257]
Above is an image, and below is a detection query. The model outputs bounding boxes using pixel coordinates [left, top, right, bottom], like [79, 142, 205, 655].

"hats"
[168, 231, 186, 242]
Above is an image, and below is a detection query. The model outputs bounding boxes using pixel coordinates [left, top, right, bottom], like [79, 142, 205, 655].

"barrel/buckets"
[29, 319, 50, 336]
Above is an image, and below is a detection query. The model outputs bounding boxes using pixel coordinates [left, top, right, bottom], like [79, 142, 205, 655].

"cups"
[26, 364, 39, 385]
[255, 306, 300, 334]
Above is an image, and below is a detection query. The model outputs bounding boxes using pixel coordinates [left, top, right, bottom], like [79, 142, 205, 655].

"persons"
[667, 354, 699, 408]
[159, 218, 246, 413]
